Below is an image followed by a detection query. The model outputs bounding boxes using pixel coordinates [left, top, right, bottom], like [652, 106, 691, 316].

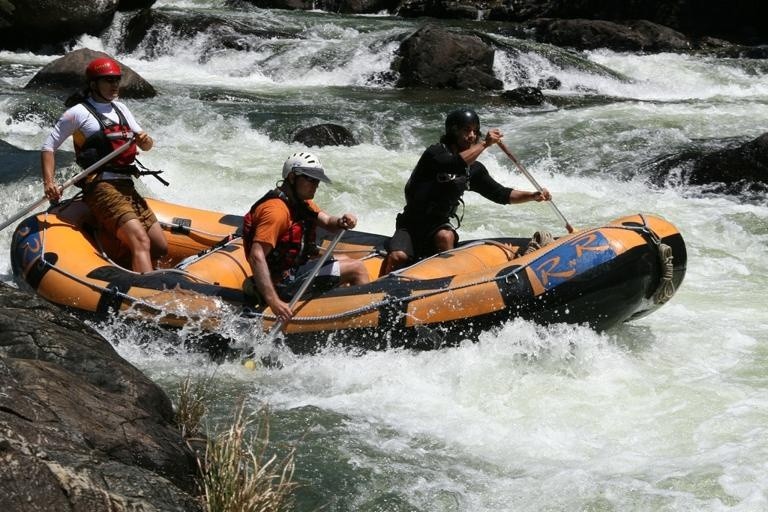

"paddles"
[240, 217, 348, 366]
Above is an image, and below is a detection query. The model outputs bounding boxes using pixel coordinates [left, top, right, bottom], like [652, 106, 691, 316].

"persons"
[243, 152, 370, 325]
[385, 107, 552, 275]
[42, 58, 169, 274]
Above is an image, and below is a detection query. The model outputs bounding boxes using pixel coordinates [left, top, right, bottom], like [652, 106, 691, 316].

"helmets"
[86, 57, 123, 76]
[282, 152, 331, 182]
[446, 108, 480, 135]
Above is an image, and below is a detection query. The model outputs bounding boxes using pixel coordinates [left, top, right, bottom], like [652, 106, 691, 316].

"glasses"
[300, 174, 316, 182]
[103, 78, 120, 82]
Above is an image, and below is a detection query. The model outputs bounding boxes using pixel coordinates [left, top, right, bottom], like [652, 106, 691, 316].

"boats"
[9, 195, 687, 368]
